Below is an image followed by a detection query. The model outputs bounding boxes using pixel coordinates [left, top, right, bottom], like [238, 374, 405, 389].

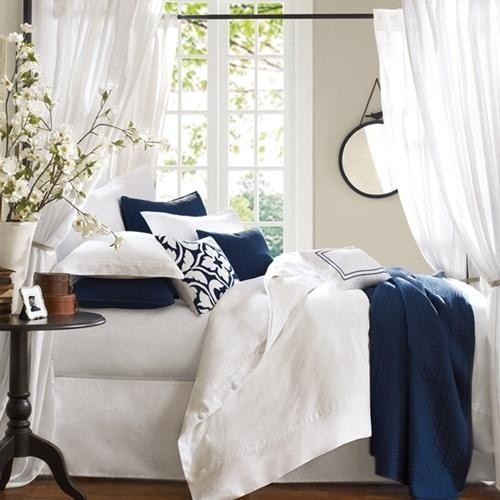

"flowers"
[0, 20, 173, 251]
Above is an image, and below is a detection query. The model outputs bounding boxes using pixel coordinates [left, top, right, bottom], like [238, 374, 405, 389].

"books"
[0, 268, 16, 320]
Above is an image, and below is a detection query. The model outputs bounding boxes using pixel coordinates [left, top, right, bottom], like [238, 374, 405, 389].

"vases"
[0, 222, 38, 316]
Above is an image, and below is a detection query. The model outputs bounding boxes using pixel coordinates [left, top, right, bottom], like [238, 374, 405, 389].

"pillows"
[119, 189, 208, 233]
[298, 245, 392, 291]
[140, 207, 246, 235]
[154, 234, 242, 318]
[193, 227, 274, 281]
[49, 229, 187, 281]
[70, 277, 178, 311]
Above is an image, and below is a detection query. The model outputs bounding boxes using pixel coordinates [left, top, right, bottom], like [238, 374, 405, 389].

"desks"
[0, 305, 108, 500]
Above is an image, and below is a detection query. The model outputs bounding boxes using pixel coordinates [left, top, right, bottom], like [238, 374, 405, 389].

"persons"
[29, 296, 41, 312]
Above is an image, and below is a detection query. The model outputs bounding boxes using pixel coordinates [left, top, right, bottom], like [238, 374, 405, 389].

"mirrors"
[337, 118, 397, 201]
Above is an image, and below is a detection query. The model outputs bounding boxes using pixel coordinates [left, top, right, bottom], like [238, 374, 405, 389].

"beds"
[0, 241, 498, 487]
